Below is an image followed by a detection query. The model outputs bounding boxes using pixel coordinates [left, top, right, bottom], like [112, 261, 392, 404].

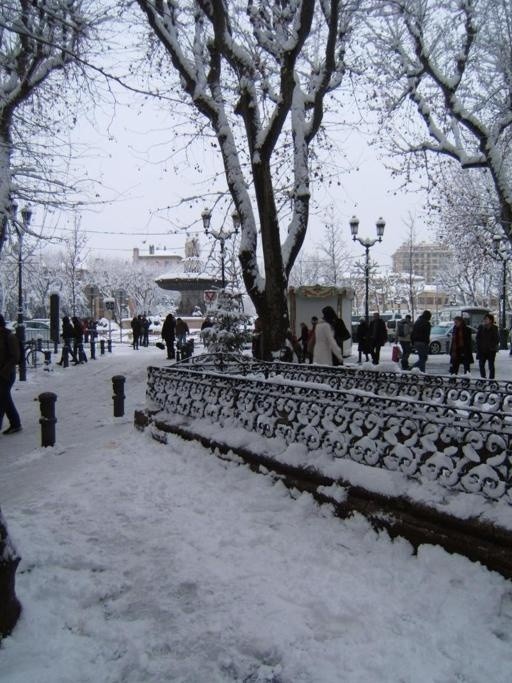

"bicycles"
[24, 336, 46, 368]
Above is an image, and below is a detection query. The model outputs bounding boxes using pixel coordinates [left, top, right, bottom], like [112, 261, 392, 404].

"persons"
[355, 317, 368, 365]
[448, 315, 475, 376]
[296, 304, 351, 367]
[409, 310, 430, 375]
[477, 312, 499, 378]
[393, 312, 413, 371]
[60, 314, 214, 369]
[367, 312, 387, 363]
[0, 315, 26, 436]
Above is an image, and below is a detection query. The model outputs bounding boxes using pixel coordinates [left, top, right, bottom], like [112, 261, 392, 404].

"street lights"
[492, 233, 511, 350]
[349, 214, 386, 321]
[10, 198, 33, 381]
[201, 206, 241, 293]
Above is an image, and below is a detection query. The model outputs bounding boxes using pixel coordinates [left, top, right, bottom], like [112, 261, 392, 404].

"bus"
[351, 312, 404, 342]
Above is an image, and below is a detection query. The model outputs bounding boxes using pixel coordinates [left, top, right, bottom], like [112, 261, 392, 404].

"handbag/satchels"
[392, 346, 401, 362]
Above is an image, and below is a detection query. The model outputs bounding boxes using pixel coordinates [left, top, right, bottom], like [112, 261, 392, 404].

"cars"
[413, 321, 478, 353]
[5, 317, 64, 342]
